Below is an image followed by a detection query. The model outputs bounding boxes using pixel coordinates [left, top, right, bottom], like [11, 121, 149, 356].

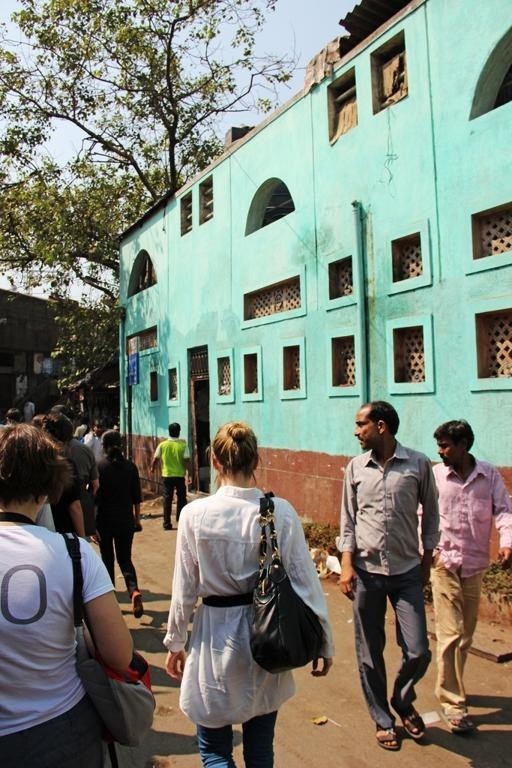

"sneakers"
[131, 591, 144, 619]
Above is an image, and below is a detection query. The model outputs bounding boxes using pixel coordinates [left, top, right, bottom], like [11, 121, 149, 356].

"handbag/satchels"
[75, 625, 156, 748]
[245, 560, 320, 675]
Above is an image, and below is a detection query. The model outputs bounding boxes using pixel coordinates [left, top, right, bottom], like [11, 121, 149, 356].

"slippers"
[438, 707, 476, 733]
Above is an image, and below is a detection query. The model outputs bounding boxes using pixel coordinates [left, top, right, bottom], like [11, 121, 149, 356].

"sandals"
[375, 724, 401, 751]
[390, 699, 426, 740]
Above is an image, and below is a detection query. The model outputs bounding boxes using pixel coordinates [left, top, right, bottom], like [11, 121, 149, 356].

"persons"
[148, 422, 195, 530]
[0, 419, 136, 767]
[0, 386, 147, 620]
[416, 418, 512, 737]
[336, 400, 440, 752]
[162, 421, 334, 766]
